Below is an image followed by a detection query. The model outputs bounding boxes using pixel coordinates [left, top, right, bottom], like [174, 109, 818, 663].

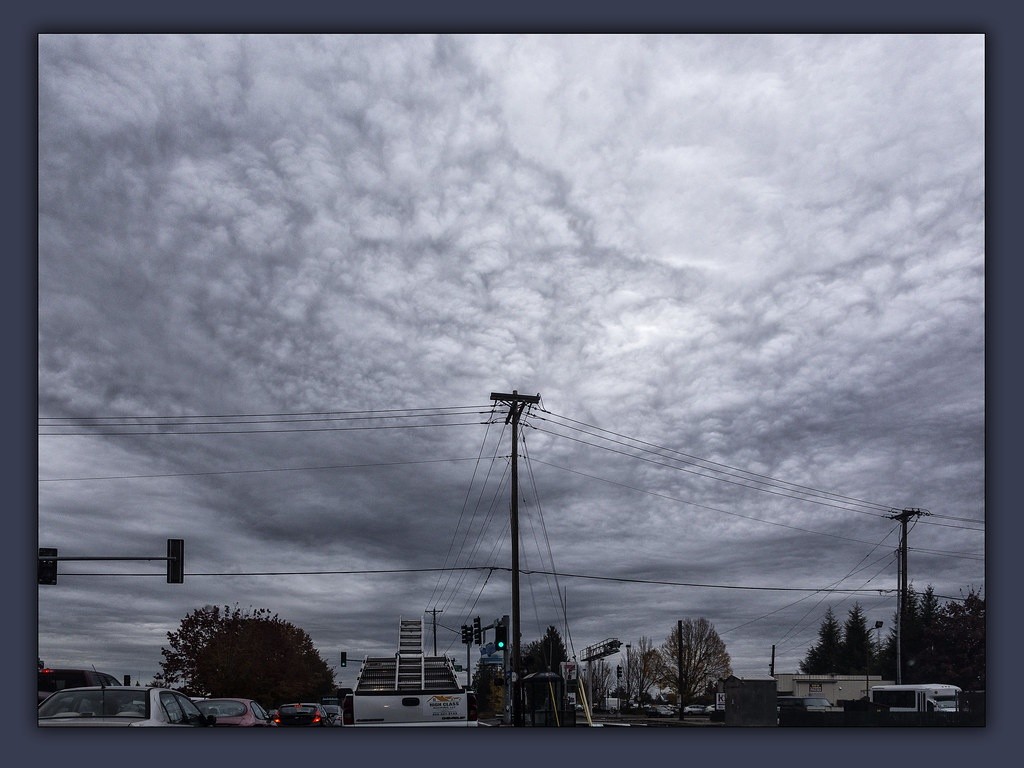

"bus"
[871, 684, 962, 713]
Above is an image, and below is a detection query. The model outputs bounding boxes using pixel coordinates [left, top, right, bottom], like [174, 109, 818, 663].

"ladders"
[395, 615, 425, 691]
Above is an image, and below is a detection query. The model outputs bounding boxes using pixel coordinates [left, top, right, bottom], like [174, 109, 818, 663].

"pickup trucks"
[342, 614, 479, 727]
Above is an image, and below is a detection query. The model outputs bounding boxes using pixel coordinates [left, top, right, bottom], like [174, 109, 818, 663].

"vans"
[777, 696, 844, 724]
[38, 668, 123, 705]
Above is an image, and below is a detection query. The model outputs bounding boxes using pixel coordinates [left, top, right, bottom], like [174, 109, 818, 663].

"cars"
[323, 705, 344, 727]
[645, 704, 715, 718]
[274, 702, 332, 727]
[38, 685, 217, 727]
[194, 697, 279, 726]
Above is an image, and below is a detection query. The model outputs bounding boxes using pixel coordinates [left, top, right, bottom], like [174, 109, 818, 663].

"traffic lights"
[341, 652, 346, 667]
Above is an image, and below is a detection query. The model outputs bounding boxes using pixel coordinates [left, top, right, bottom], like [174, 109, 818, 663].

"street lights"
[495, 627, 508, 651]
[865, 621, 884, 697]
